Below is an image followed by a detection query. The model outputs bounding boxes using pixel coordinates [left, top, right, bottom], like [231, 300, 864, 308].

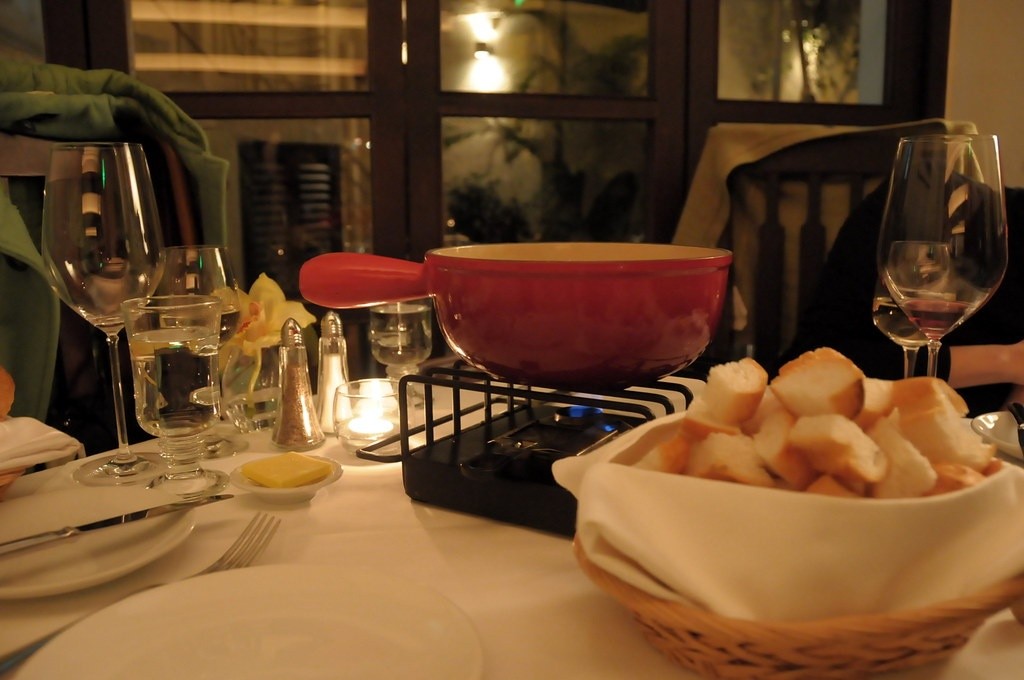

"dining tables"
[0, 378, 1024, 680]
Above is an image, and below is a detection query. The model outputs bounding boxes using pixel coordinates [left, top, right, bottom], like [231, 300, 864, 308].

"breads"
[660, 346, 1004, 503]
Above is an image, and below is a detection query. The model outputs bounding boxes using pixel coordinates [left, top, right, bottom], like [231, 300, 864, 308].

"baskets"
[572, 532, 1024, 680]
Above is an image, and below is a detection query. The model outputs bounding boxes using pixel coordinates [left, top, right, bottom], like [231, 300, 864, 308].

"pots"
[299, 243, 732, 391]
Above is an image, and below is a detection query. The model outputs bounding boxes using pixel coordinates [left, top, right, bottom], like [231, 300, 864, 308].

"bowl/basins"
[230, 454, 344, 504]
[551, 412, 1024, 623]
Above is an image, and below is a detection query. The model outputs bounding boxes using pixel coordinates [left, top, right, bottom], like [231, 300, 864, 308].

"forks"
[1, 511, 281, 676]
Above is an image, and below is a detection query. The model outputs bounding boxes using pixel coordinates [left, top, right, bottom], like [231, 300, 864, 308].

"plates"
[12, 564, 484, 680]
[0, 487, 196, 601]
[971, 411, 1024, 460]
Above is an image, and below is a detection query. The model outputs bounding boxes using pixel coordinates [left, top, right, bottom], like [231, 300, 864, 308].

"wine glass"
[121, 295, 230, 498]
[42, 141, 167, 487]
[876, 133, 1009, 382]
[157, 244, 248, 460]
[872, 242, 956, 379]
[371, 295, 434, 408]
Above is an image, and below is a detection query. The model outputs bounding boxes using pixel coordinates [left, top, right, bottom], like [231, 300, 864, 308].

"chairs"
[1, 127, 200, 443]
[716, 119, 948, 377]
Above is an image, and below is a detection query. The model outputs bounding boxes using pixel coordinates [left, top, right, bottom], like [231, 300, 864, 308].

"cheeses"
[242, 451, 332, 487]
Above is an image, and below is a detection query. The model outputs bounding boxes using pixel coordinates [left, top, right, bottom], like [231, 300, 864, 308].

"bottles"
[315, 310, 353, 437]
[270, 317, 327, 451]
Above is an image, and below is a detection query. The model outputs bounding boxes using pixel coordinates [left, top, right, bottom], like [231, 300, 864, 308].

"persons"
[775, 150, 1024, 427]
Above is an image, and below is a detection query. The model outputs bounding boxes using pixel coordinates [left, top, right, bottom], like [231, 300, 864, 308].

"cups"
[333, 378, 415, 455]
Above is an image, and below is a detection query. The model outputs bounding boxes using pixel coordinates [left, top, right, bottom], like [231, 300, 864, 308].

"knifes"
[0, 494, 234, 554]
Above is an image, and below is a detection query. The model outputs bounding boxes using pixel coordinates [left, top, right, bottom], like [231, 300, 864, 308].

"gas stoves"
[354, 356, 697, 537]
